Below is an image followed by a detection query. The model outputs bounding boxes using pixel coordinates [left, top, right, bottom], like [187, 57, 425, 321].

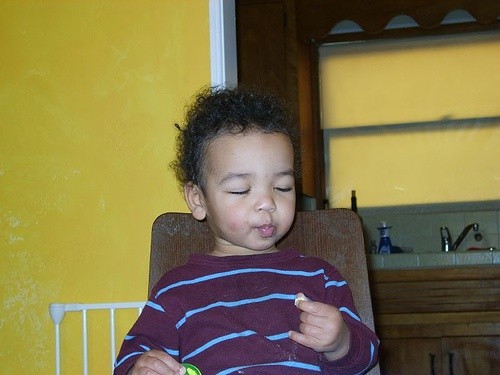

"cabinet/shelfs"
[235, 0, 326, 200]
[365, 251, 500, 375]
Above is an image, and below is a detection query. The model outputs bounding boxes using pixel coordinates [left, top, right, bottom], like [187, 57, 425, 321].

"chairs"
[147, 208, 380, 375]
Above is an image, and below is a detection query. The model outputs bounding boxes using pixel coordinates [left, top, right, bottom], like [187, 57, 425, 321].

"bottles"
[322, 198, 330, 209]
[350, 189, 363, 233]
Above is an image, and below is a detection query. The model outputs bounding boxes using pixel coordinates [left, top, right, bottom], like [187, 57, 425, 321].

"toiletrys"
[377, 221, 392, 255]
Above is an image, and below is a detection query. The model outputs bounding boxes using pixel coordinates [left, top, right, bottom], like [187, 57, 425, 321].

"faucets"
[453, 223, 479, 251]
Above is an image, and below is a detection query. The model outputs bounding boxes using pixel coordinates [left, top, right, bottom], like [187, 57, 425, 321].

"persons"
[113, 82, 380, 375]
